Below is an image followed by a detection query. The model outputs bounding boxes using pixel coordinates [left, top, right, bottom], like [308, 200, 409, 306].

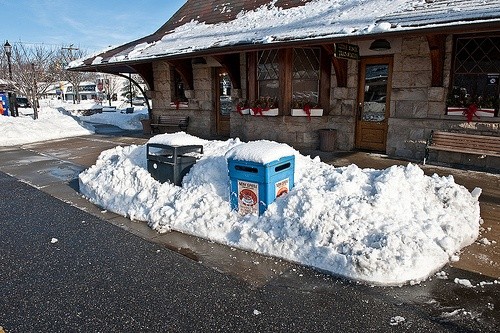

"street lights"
[4, 40, 18, 116]
[31, 63, 39, 108]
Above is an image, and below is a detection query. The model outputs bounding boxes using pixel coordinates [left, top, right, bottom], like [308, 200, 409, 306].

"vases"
[239, 109, 326, 116]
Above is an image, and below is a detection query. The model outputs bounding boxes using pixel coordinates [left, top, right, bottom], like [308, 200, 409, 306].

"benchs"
[421, 130, 500, 172]
[149, 116, 189, 133]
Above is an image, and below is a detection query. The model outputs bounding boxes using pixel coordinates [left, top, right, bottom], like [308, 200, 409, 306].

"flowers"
[236, 93, 321, 110]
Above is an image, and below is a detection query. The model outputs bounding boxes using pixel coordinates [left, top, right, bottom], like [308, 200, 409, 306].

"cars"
[15, 97, 31, 109]
[123, 93, 148, 107]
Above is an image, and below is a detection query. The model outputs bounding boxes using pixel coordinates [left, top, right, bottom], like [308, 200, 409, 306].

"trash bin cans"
[226, 156, 294, 219]
[145, 143, 202, 188]
